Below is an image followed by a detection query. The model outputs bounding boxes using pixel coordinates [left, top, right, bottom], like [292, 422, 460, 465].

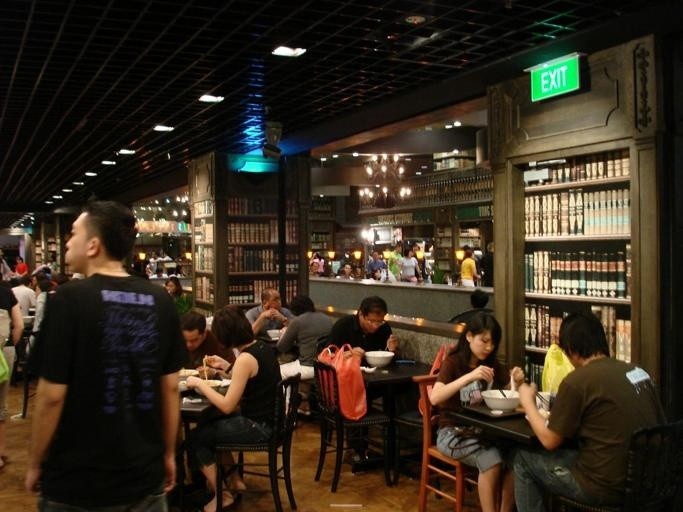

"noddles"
[202, 354, 216, 385]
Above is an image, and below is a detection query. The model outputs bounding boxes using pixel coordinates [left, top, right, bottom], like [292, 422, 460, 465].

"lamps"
[357, 154, 412, 206]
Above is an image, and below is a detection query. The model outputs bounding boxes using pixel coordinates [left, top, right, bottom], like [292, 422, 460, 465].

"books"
[524, 152, 630, 392]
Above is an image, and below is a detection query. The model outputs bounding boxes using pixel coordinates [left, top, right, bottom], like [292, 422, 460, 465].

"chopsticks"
[491, 377, 506, 398]
[533, 390, 549, 407]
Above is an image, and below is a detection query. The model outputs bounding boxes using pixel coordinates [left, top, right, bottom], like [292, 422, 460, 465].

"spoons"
[506, 374, 515, 397]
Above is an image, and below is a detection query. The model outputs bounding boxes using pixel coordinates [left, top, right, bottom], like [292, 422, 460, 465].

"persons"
[1, 256, 70, 420]
[309, 241, 494, 288]
[165, 277, 293, 495]
[316, 297, 400, 465]
[187, 304, 282, 512]
[134, 251, 181, 278]
[431, 313, 515, 512]
[512, 312, 662, 512]
[26, 202, 190, 512]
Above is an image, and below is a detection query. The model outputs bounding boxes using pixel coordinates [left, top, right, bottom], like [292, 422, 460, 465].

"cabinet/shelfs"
[47, 241, 56, 253]
[358, 196, 493, 283]
[35, 245, 41, 263]
[227, 190, 299, 310]
[190, 197, 216, 316]
[312, 195, 337, 256]
[505, 137, 640, 392]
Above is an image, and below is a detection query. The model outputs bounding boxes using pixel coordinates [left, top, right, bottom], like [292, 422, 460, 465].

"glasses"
[365, 314, 387, 327]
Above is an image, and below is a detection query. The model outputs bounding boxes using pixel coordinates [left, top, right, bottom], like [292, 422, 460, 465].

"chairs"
[210, 372, 301, 512]
[394, 412, 439, 484]
[312, 360, 393, 493]
[556, 423, 682, 511]
[412, 375, 477, 511]
[12, 329, 35, 419]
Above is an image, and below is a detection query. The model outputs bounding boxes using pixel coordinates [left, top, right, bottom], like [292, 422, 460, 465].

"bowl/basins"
[266, 329, 284, 339]
[192, 379, 230, 397]
[174, 369, 199, 391]
[534, 391, 556, 420]
[364, 350, 394, 368]
[480, 389, 522, 413]
[22, 316, 34, 324]
